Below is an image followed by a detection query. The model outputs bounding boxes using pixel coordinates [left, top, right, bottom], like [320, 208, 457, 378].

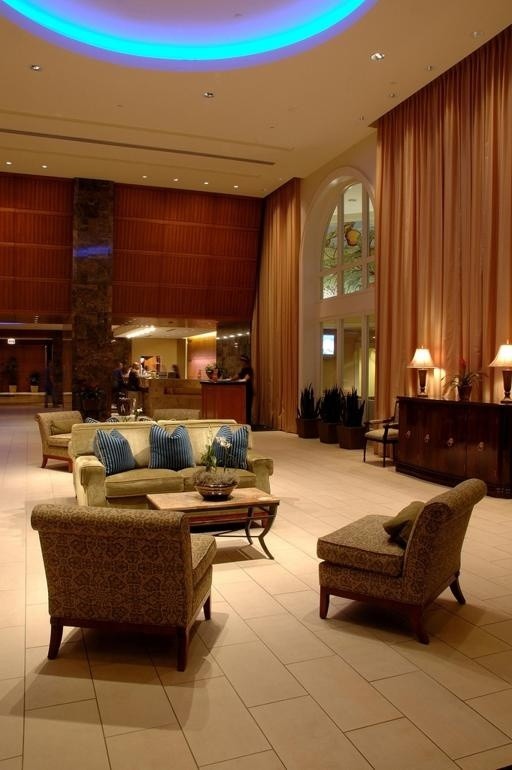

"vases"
[194, 484, 237, 499]
[83, 397, 102, 410]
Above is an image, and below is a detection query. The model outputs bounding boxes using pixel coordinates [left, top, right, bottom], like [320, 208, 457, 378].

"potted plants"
[440, 371, 488, 399]
[295, 381, 322, 438]
[318, 382, 347, 444]
[29, 370, 41, 393]
[8, 369, 17, 393]
[336, 387, 367, 450]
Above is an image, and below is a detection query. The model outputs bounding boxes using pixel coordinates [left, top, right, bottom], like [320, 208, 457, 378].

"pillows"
[148, 422, 195, 470]
[210, 424, 250, 471]
[49, 417, 82, 436]
[85, 416, 120, 424]
[383, 499, 425, 550]
[92, 427, 137, 476]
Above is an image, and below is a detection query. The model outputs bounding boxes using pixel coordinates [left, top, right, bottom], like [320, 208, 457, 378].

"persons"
[227, 355, 254, 424]
[6, 357, 18, 391]
[45, 360, 60, 408]
[128, 362, 146, 414]
[172, 364, 180, 378]
[112, 362, 128, 405]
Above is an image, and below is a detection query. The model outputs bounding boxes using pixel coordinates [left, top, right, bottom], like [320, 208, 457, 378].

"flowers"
[76, 383, 107, 400]
[134, 405, 143, 422]
[193, 434, 240, 486]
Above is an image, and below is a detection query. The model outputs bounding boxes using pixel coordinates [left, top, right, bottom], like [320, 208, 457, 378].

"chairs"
[315, 477, 488, 645]
[30, 502, 217, 673]
[361, 401, 400, 468]
[35, 411, 84, 473]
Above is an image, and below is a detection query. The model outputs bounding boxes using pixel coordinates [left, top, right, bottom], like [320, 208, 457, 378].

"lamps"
[488, 340, 512, 404]
[407, 344, 436, 396]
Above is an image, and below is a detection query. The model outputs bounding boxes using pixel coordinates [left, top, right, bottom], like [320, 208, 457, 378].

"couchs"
[68, 419, 275, 507]
